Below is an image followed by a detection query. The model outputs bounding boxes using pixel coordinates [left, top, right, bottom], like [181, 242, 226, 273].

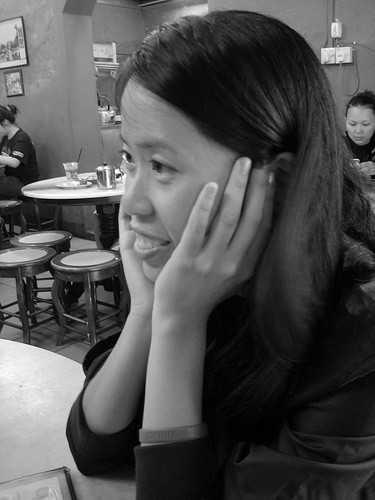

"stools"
[9, 230, 80, 311]
[0, 199, 26, 250]
[0, 246, 60, 345]
[18, 195, 60, 230]
[50, 248, 130, 348]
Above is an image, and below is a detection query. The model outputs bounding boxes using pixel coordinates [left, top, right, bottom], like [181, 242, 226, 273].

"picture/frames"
[3, 69, 25, 98]
[0, 15, 30, 70]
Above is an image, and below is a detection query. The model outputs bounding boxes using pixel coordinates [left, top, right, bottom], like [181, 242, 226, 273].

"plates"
[56, 182, 92, 189]
[86, 179, 97, 184]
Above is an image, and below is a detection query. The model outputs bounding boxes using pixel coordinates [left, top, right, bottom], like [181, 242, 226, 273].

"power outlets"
[336, 47, 353, 64]
[321, 47, 336, 64]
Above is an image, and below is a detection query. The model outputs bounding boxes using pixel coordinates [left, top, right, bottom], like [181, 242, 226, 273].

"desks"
[0, 338, 136, 500]
[21, 169, 125, 308]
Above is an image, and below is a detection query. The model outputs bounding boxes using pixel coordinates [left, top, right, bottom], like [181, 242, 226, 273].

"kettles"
[97, 96, 115, 125]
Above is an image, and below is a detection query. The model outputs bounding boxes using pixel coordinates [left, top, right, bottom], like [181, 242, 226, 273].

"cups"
[96, 161, 122, 189]
[62, 161, 79, 181]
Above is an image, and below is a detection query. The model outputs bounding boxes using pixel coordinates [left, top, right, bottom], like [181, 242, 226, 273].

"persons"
[0, 103, 40, 201]
[66, 10, 374, 500]
[338, 88, 374, 184]
